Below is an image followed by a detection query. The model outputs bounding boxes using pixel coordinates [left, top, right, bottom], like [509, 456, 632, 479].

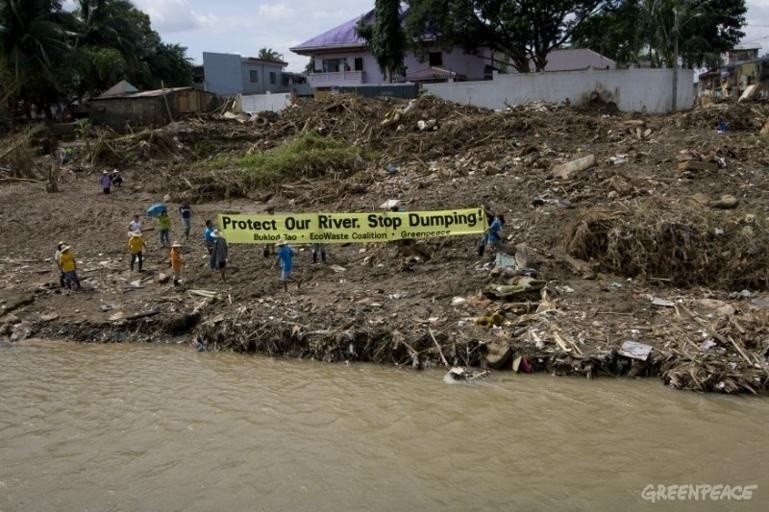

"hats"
[132, 229, 142, 237]
[210, 229, 223, 238]
[113, 168, 120, 174]
[57, 241, 71, 253]
[103, 170, 109, 175]
[274, 239, 288, 248]
[171, 240, 182, 247]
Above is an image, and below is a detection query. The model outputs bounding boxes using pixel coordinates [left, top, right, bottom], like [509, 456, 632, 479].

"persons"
[127, 201, 228, 287]
[54, 241, 69, 288]
[264, 207, 277, 255]
[310, 243, 327, 265]
[483, 214, 506, 256]
[99, 170, 111, 194]
[472, 204, 495, 256]
[60, 245, 84, 289]
[109, 169, 122, 187]
[271, 239, 293, 293]
